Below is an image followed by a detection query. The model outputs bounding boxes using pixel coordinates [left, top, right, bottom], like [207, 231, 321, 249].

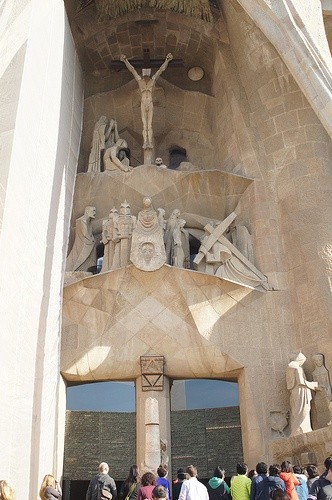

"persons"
[286, 352, 332, 435]
[66, 197, 262, 288]
[119, 457, 332, 500]
[86, 462, 117, 500]
[88, 53, 173, 172]
[0, 480, 14, 500]
[39, 474, 62, 500]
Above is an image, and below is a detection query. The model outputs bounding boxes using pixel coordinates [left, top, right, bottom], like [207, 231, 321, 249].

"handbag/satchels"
[271, 488, 290, 500]
[99, 489, 112, 500]
